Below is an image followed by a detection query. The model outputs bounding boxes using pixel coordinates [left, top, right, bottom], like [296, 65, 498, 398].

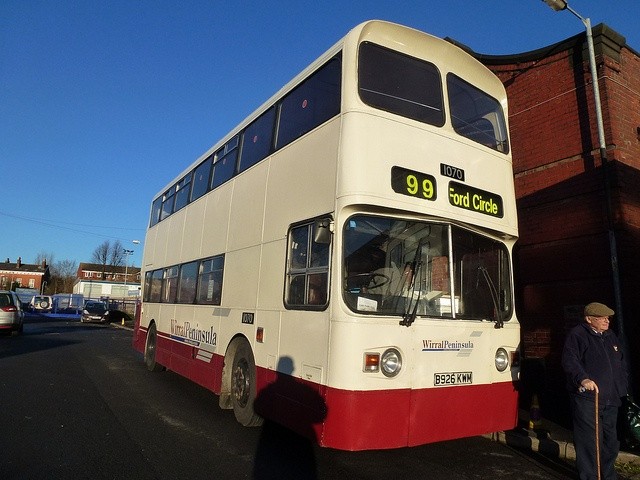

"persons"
[560, 302, 631, 480]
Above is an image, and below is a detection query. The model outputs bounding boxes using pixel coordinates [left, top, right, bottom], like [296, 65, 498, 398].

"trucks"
[52, 294, 84, 309]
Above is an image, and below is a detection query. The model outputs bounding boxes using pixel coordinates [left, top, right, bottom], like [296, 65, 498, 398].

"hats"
[583, 302, 615, 316]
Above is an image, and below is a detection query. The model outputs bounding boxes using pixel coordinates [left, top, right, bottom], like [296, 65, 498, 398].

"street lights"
[541, 0, 625, 341]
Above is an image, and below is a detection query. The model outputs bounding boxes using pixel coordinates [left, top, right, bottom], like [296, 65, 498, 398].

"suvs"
[28, 296, 52, 312]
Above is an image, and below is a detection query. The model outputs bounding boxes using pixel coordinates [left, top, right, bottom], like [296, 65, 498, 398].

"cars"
[81, 301, 110, 323]
[0, 290, 25, 331]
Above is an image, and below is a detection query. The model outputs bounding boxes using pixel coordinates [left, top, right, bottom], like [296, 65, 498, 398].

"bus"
[133, 19, 519, 451]
